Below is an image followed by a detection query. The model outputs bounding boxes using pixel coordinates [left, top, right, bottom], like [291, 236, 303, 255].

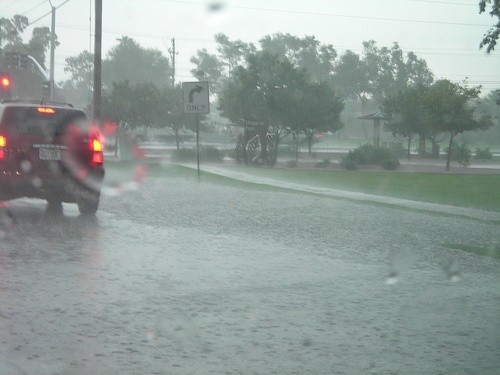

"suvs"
[0, 96, 106, 222]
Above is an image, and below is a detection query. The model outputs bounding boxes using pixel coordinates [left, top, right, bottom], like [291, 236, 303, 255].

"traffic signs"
[182, 81, 210, 114]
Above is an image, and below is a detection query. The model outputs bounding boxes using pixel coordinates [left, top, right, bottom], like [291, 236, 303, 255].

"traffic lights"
[0, 74, 12, 106]
